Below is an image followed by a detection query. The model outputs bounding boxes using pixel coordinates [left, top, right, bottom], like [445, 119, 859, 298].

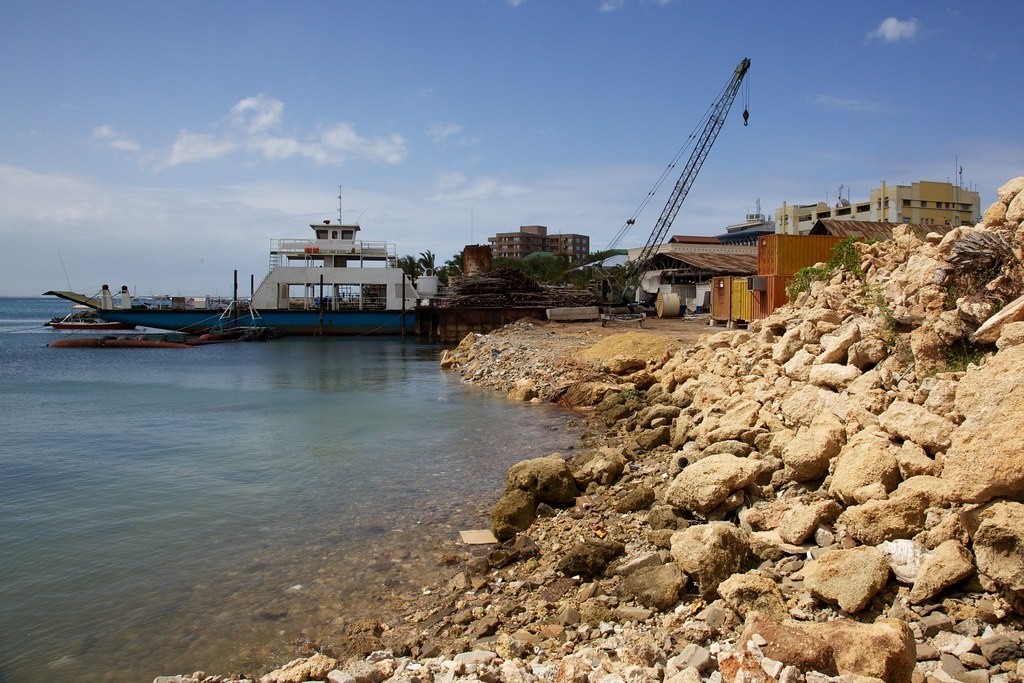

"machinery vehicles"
[586, 56, 753, 329]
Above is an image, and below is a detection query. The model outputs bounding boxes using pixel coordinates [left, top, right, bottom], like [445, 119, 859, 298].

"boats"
[71, 304, 90, 310]
[209, 300, 267, 341]
[50, 310, 137, 331]
[41, 186, 441, 340]
